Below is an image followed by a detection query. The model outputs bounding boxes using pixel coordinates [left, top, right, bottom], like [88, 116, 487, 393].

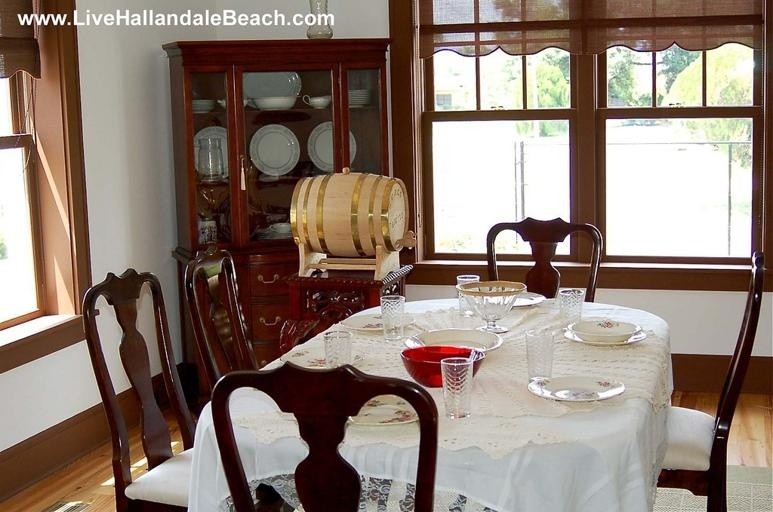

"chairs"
[82, 268, 196, 512]
[184, 247, 258, 397]
[487, 217, 603, 302]
[657, 251, 764, 512]
[211, 361, 437, 511]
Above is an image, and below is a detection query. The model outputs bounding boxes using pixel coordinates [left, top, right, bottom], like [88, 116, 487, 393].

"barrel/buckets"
[289, 166, 420, 257]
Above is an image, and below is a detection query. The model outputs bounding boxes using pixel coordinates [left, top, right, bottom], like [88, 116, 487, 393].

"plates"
[347, 89, 369, 107]
[340, 315, 414, 330]
[349, 393, 419, 425]
[243, 72, 301, 107]
[249, 124, 300, 177]
[307, 122, 356, 172]
[513, 291, 545, 308]
[404, 328, 504, 353]
[528, 376, 622, 401]
[193, 127, 228, 178]
[565, 328, 646, 347]
[572, 321, 641, 341]
[280, 348, 364, 371]
[258, 227, 295, 240]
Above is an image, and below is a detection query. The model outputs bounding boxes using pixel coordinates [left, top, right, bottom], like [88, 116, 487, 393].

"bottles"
[198, 137, 223, 180]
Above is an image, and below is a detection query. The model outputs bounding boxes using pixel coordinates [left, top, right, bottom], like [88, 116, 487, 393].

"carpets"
[653, 465, 773, 512]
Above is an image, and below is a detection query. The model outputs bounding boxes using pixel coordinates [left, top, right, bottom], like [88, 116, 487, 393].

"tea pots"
[303, 95, 331, 108]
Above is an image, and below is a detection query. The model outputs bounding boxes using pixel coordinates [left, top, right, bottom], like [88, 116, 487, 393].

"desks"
[284, 264, 414, 321]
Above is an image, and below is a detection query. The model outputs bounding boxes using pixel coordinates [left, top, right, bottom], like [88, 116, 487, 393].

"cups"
[198, 221, 218, 243]
[441, 357, 473, 418]
[559, 289, 584, 323]
[324, 331, 352, 363]
[380, 295, 405, 339]
[269, 223, 291, 231]
[456, 274, 478, 313]
[527, 332, 552, 381]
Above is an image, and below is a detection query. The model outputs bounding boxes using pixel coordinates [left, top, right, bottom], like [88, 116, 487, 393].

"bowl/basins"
[253, 95, 296, 109]
[192, 99, 215, 113]
[401, 345, 485, 387]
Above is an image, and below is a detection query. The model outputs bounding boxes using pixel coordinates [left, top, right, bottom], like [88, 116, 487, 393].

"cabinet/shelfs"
[162, 38, 393, 369]
